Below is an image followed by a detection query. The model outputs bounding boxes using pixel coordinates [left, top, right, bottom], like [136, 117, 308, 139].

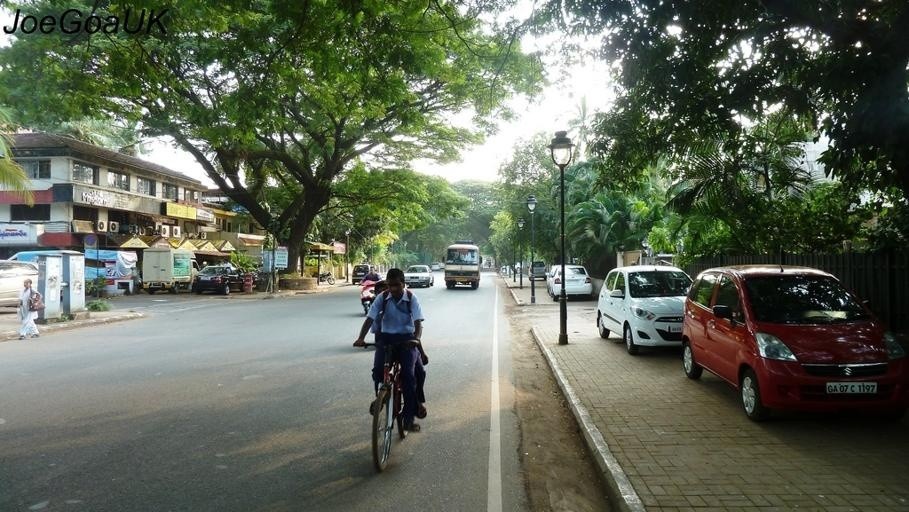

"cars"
[595, 265, 694, 358]
[195, 264, 260, 295]
[679, 245, 909, 424]
[402, 265, 436, 288]
[439, 263, 446, 271]
[542, 265, 559, 296]
[549, 265, 593, 302]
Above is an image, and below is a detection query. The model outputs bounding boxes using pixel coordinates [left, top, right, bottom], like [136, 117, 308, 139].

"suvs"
[528, 261, 547, 281]
[353, 264, 379, 286]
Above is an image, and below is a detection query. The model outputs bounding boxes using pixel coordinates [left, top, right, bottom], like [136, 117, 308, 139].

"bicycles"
[354, 340, 412, 472]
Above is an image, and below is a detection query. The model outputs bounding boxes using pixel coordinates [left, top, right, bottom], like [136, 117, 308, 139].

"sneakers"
[369, 397, 384, 417]
[19, 334, 40, 340]
[403, 406, 427, 432]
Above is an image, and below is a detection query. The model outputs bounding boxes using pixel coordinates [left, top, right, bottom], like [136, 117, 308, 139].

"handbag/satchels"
[29, 289, 46, 312]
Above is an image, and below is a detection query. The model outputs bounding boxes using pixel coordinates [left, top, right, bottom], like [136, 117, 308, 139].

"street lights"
[546, 128, 577, 347]
[506, 233, 512, 278]
[523, 193, 540, 304]
[515, 217, 526, 289]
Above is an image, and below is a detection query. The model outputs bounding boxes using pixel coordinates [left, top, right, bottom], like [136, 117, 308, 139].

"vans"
[0, 261, 41, 310]
[7, 250, 85, 264]
[429, 261, 440, 272]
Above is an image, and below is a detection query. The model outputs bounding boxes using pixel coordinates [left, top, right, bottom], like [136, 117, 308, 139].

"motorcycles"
[313, 269, 338, 285]
[357, 280, 375, 316]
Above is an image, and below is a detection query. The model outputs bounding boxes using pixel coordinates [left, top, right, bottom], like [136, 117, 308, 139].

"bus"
[441, 240, 484, 294]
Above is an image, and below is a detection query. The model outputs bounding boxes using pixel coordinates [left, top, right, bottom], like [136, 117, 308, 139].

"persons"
[355, 268, 424, 433]
[360, 266, 382, 282]
[201, 258, 232, 271]
[18, 279, 42, 339]
[371, 280, 429, 419]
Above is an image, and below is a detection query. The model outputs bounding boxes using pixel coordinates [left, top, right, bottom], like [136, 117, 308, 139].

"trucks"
[142, 247, 200, 294]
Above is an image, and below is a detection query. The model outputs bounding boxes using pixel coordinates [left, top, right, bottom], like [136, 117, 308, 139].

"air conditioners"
[96, 219, 183, 238]
